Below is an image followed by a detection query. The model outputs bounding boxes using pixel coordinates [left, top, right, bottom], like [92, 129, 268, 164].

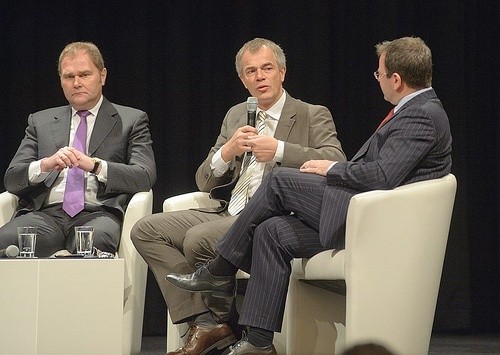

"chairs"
[0, 187, 155, 355]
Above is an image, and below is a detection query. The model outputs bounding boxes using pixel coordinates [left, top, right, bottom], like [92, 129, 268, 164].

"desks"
[0, 257, 126, 355]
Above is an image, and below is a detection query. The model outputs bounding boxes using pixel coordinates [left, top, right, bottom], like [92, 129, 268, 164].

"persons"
[130, 38, 349, 355]
[0, 42, 157, 260]
[163, 37, 453, 355]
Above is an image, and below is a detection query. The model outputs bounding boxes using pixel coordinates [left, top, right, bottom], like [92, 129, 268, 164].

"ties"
[227, 111, 267, 217]
[376, 107, 394, 129]
[61, 110, 92, 219]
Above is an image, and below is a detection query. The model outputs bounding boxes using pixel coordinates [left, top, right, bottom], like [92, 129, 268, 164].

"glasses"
[373, 70, 393, 79]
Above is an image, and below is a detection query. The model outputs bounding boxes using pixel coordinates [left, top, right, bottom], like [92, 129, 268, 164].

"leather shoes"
[165, 322, 237, 355]
[225, 330, 278, 355]
[165, 260, 239, 298]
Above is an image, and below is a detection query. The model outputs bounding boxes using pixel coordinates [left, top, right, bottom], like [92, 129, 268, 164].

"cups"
[16, 226, 37, 258]
[74, 226, 95, 256]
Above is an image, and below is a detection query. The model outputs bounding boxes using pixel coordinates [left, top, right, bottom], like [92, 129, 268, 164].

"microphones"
[246, 97, 259, 156]
[0, 245, 20, 259]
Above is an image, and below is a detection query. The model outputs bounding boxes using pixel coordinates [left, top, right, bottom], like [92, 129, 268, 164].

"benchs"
[162, 172, 458, 355]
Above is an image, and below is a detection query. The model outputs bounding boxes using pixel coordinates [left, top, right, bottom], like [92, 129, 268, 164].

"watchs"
[89, 157, 101, 173]
